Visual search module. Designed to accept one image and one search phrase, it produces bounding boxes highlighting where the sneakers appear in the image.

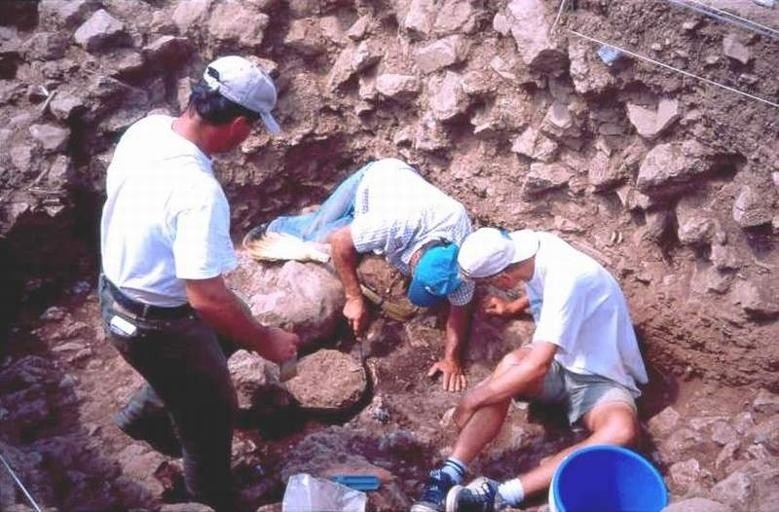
[241,222,278,255]
[118,396,184,458]
[445,474,504,512]
[408,467,459,512]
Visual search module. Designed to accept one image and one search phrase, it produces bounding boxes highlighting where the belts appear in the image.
[102,274,195,320]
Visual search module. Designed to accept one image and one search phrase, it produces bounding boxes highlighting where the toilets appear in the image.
[548,445,669,512]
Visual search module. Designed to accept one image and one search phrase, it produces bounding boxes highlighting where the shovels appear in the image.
[350,330,364,367]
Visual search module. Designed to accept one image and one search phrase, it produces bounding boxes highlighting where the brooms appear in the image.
[250,230,332,266]
[359,283,420,324]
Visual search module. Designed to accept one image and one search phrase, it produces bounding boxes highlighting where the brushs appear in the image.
[279,322,298,383]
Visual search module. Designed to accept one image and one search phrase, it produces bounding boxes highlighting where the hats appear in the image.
[457,226,539,279]
[407,242,463,307]
[202,55,282,134]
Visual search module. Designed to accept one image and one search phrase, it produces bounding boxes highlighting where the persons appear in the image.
[100,53,298,509]
[412,228,649,512]
[263,156,473,391]
